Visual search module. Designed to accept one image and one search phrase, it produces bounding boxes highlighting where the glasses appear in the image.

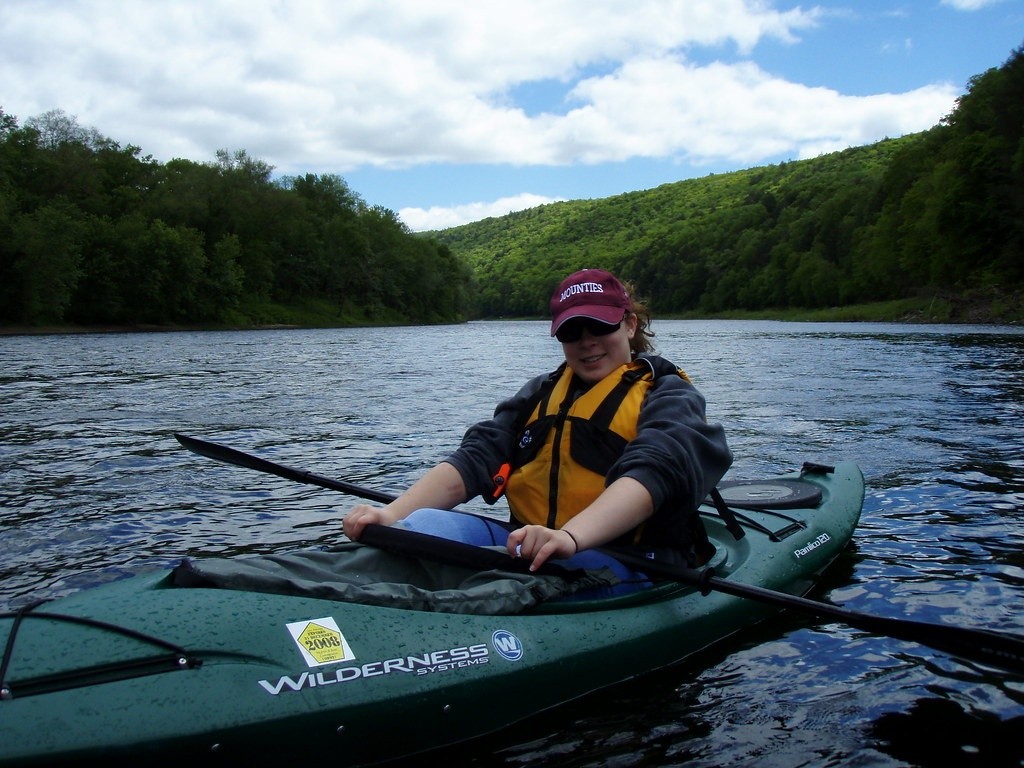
[553,318,626,344]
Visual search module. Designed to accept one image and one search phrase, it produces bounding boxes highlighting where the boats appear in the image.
[1,454,870,768]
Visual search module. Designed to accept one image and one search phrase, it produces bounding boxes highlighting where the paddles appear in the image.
[172,431,1024,670]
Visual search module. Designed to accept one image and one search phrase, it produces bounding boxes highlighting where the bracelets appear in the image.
[561,530,578,556]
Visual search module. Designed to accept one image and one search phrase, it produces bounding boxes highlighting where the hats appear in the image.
[551,269,633,337]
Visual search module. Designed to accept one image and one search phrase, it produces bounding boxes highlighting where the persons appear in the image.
[342,268,734,597]
